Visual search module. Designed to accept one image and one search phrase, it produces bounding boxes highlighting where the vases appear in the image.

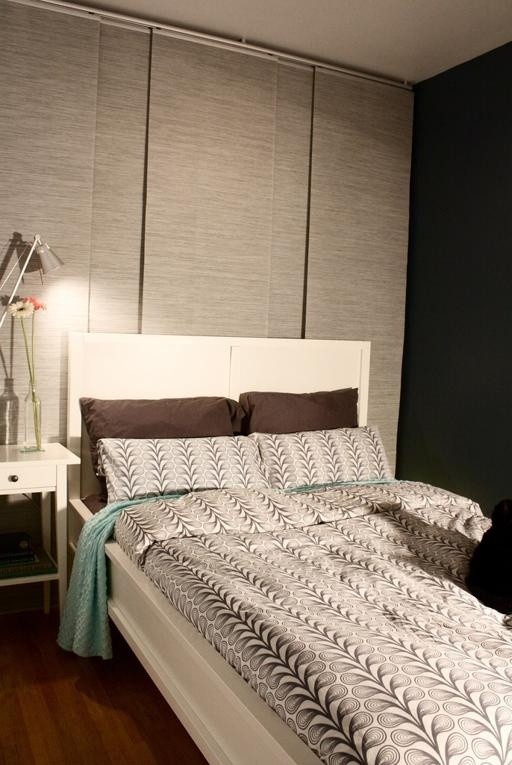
[18,381,41,452]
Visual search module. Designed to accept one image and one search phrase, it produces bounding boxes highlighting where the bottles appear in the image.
[20,380,42,452]
[0,378,18,445]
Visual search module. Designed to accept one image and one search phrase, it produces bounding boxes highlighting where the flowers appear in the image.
[9,297,47,450]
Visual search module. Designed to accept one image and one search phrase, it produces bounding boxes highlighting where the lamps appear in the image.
[0,234,64,329]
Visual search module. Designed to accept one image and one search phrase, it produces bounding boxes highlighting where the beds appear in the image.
[66,333,512,765]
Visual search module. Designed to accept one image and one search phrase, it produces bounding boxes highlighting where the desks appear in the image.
[0,443,82,624]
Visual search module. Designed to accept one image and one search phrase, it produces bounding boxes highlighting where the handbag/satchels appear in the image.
[466,498,512,614]
